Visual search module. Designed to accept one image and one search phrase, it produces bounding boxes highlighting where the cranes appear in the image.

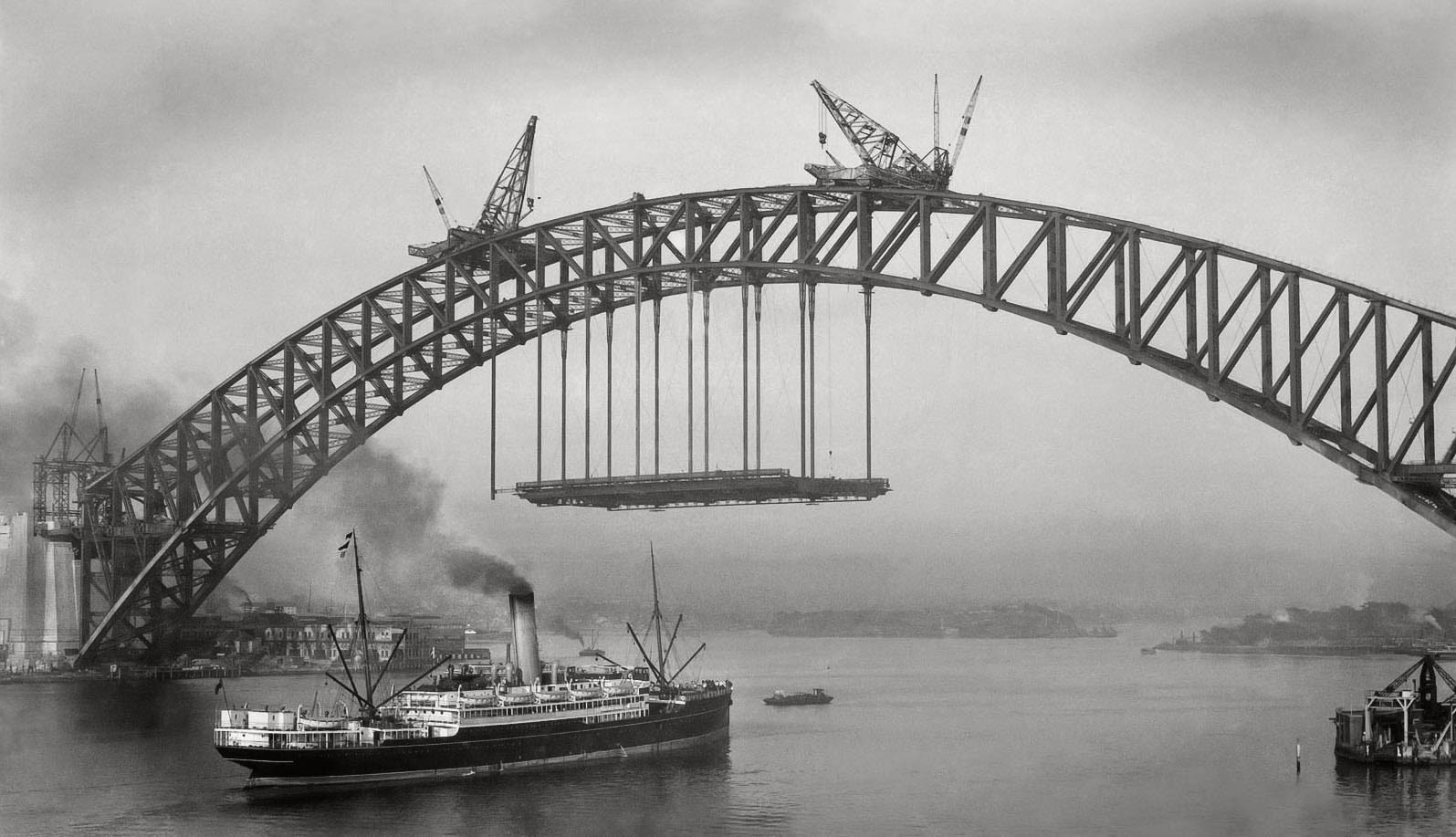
[33,368,117,527]
[407,116,562,277]
[804,72,983,212]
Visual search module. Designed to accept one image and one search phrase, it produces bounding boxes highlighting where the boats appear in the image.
[764,690,834,706]
[212,526,732,789]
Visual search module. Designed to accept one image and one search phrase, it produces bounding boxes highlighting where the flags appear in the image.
[215,677,222,695]
[338,540,349,550]
[345,531,352,538]
